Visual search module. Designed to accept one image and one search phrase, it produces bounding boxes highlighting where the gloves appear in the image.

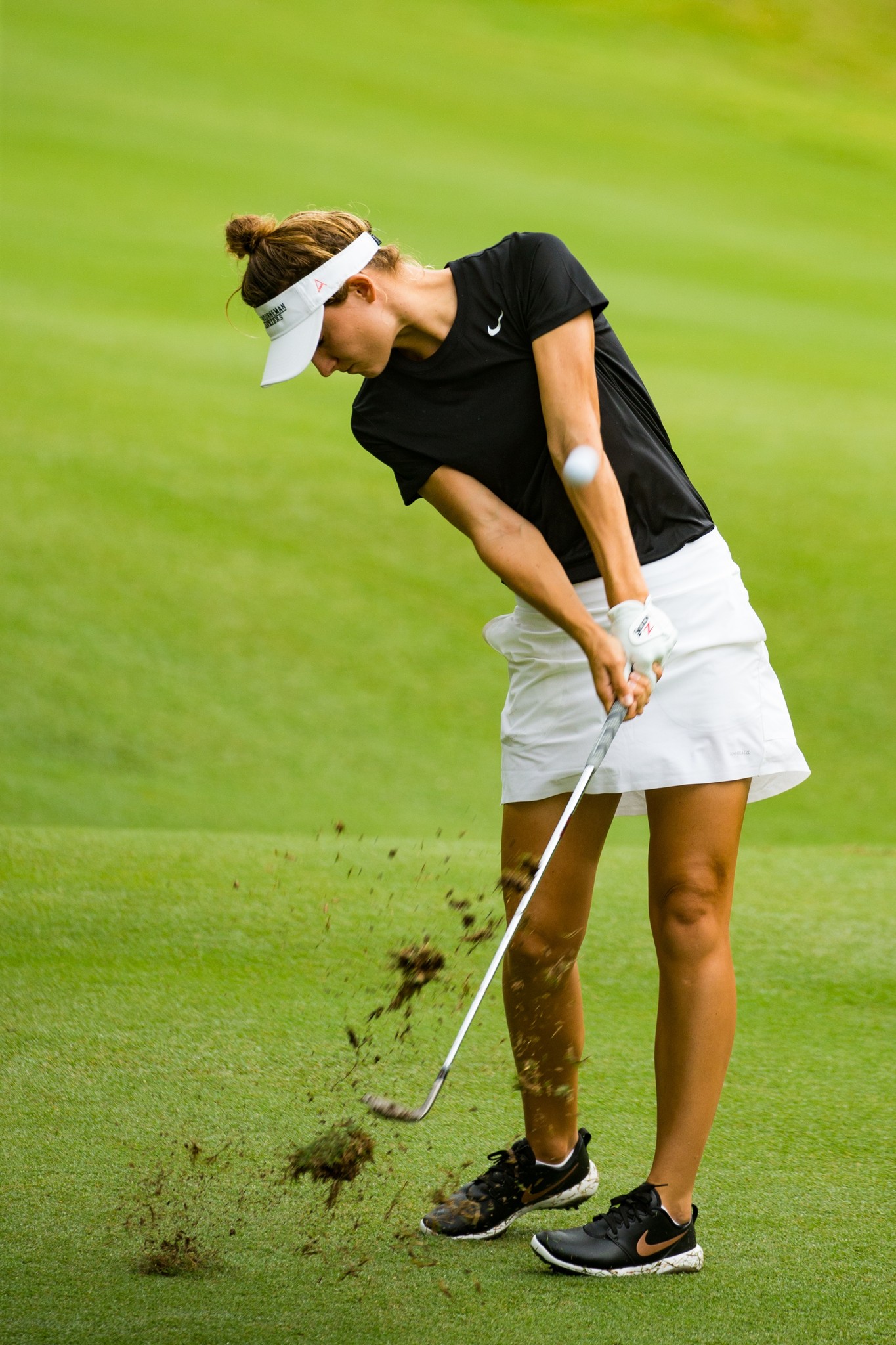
[607,600,664,692]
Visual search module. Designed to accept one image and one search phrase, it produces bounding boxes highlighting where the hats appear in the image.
[254,231,382,388]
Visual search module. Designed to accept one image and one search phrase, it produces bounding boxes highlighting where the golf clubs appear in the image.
[365,658,637,1123]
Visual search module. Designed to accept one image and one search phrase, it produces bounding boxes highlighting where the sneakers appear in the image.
[530,1182,705,1278]
[420,1126,599,1241]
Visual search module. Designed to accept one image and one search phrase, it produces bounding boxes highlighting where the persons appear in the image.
[224,203,808,1279]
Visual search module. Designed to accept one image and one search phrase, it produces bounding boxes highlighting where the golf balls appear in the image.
[564,444,602,485]
